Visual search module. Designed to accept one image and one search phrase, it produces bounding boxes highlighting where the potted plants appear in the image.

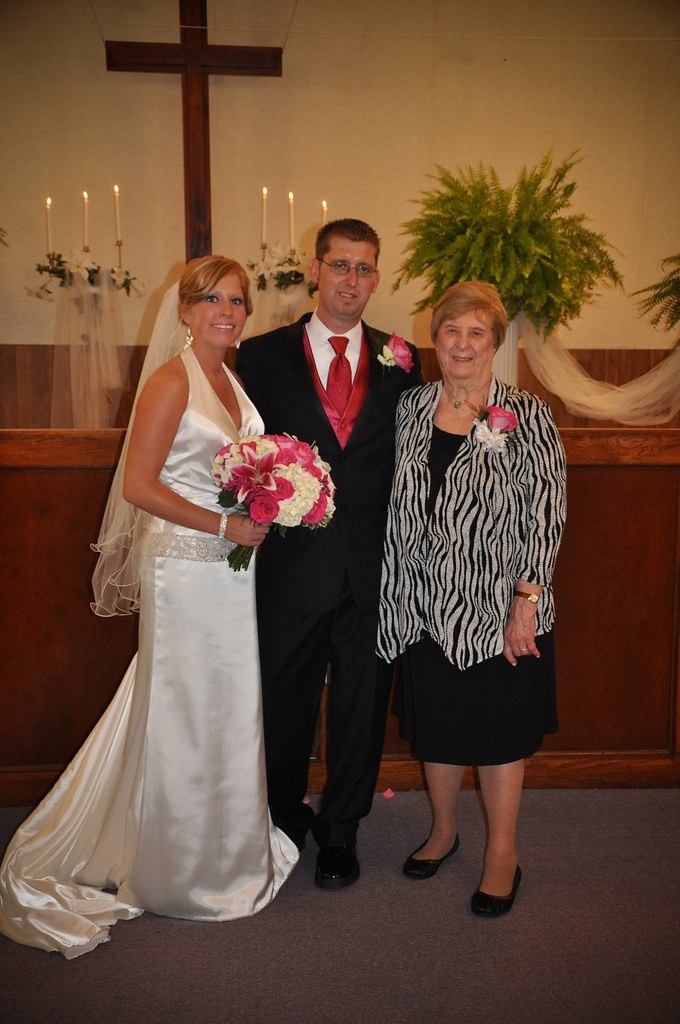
[388,146,628,385]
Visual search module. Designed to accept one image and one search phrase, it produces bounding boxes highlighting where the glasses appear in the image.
[318,258,377,277]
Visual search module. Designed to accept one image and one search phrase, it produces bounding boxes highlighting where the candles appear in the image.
[115,193,121,242]
[262,195,267,243]
[289,200,295,244]
[83,198,89,248]
[45,205,52,253]
[322,207,327,225]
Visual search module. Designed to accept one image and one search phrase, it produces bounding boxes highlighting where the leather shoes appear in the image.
[470,858,522,917]
[278,820,305,853]
[313,842,359,889]
[403,829,461,879]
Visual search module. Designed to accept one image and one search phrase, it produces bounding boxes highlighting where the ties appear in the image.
[325,336,351,418]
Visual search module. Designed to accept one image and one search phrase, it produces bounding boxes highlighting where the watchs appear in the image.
[514,591,540,603]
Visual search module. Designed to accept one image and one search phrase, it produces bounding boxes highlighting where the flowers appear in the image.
[210,433,337,572]
[463,398,523,457]
[376,332,415,376]
[248,243,319,299]
[23,252,147,303]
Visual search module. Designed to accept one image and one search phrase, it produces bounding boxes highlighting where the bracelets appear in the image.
[218,513,227,539]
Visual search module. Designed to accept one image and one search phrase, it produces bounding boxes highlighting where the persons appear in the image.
[0,255,301,959]
[376,280,565,915]
[237,218,421,887]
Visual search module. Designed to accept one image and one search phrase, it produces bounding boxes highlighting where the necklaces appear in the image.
[444,379,491,407]
